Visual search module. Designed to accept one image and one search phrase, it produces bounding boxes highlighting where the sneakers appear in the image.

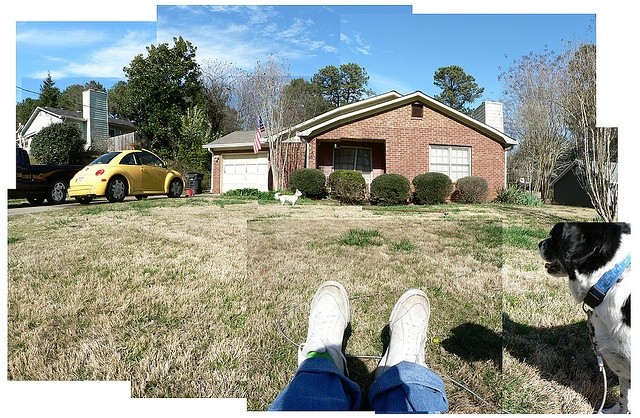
[373,289,431,378]
[297,281,350,376]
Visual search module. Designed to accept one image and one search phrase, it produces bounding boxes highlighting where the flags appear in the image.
[252,115,265,154]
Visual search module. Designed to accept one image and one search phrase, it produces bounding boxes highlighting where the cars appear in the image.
[67,148,184,203]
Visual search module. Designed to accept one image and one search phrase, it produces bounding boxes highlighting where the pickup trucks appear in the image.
[8,148,84,204]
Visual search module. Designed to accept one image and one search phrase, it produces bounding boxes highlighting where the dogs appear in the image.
[538,221,631,414]
[274,188,303,206]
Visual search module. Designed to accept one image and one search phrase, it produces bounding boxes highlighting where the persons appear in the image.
[267,280,450,414]
